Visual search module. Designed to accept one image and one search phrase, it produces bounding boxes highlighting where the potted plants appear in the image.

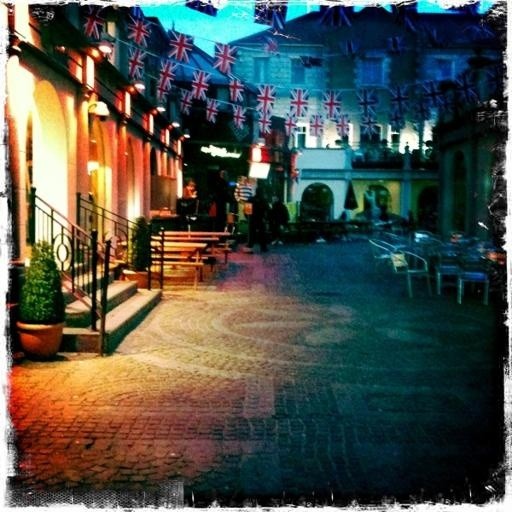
[122,216,152,289]
[16,240,65,363]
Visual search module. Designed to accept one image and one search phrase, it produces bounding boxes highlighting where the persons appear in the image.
[203,176,239,243]
[235,175,255,221]
[244,189,268,253]
[183,177,197,200]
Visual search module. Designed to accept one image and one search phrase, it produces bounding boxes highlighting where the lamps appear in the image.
[87,38,196,143]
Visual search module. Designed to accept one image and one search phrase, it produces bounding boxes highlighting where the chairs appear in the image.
[365,220,500,308]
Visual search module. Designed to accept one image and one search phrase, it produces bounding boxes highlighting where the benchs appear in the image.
[121,231,233,287]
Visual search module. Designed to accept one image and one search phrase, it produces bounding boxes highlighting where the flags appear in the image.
[309,89,349,136]
[356,72,478,140]
[185,1,496,55]
[483,66,500,94]
[129,48,309,136]
[85,5,238,75]
